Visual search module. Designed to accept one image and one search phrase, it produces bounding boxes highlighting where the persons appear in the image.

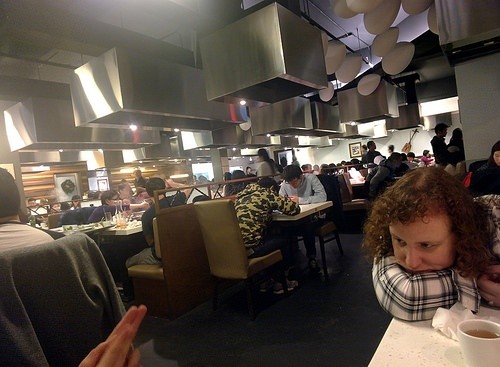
[117,177,186,291]
[235,177,300,294]
[446,128,465,162]
[0,166,68,259]
[26,136,434,215]
[84,189,150,224]
[359,165,500,321]
[79,304,148,367]
[275,164,327,275]
[430,123,450,166]
[463,140,500,197]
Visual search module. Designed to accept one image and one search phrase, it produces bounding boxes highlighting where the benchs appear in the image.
[127,195,241,319]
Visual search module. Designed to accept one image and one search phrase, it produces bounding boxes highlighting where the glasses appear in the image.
[109,196,119,201]
[285,175,300,184]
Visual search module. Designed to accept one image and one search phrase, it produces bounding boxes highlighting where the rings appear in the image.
[487,299,496,307]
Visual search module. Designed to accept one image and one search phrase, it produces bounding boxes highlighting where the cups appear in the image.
[104,212,112,222]
[116,214,127,229]
[457,319,500,367]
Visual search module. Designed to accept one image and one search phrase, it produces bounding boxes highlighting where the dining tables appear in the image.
[368,302,500,367]
[27,218,142,267]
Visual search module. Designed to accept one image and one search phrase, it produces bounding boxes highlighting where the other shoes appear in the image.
[306,252,317,263]
[260,280,298,294]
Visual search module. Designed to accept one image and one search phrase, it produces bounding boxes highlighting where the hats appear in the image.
[374,156,385,165]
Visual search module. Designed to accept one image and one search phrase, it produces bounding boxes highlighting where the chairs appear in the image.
[194,199,288,321]
[0,232,135,367]
[470,160,489,174]
[338,173,370,234]
[286,174,343,277]
[33,206,98,229]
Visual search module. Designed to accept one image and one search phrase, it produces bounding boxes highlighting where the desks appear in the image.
[349,179,365,200]
[272,201,333,282]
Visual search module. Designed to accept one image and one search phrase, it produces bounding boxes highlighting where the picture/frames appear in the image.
[349,142,362,158]
